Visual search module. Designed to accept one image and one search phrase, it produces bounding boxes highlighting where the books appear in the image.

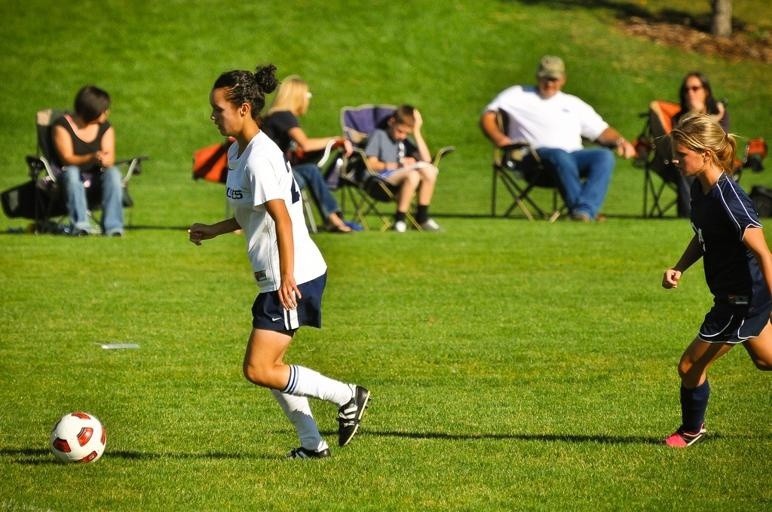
[380,162,439,179]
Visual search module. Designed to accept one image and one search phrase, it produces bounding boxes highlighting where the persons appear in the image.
[480,55,636,224]
[50,87,125,237]
[672,71,729,217]
[188,63,371,460]
[259,75,355,234]
[663,113,772,448]
[365,106,441,233]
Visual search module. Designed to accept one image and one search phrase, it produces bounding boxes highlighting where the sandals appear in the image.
[328,224,352,235]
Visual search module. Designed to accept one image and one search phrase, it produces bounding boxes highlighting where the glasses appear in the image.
[680,84,702,93]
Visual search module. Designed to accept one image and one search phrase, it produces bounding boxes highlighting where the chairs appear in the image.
[632,100,728,219]
[338,103,456,233]
[490,107,587,224]
[26,107,150,238]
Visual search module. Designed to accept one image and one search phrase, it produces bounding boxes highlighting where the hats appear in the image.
[535,54,564,79]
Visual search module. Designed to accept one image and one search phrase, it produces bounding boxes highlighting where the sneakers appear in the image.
[338,384,370,447]
[285,447,331,460]
[571,211,606,224]
[395,218,441,233]
[665,422,708,448]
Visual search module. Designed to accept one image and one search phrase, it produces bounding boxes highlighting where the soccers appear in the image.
[49,412,106,466]
